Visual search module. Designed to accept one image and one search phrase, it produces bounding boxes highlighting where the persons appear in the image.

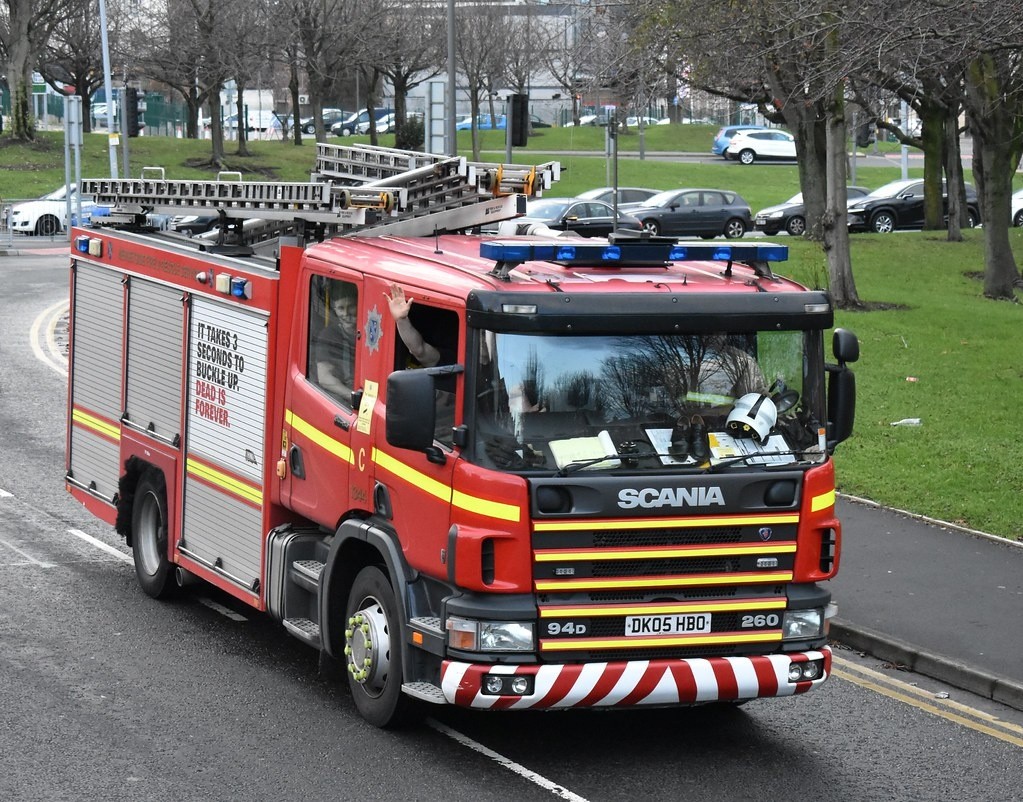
[383,282,548,412]
[660,330,774,415]
[316,288,357,402]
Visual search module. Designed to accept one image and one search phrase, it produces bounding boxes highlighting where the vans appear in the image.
[329,108,397,138]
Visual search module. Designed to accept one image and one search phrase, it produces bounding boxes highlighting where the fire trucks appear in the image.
[63,144,860,731]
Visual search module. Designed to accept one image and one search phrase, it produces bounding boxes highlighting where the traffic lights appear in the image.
[855,112,876,148]
[120,83,146,137]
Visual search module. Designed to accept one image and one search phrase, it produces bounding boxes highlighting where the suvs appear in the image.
[726,129,798,165]
[712,125,786,161]
[619,188,753,240]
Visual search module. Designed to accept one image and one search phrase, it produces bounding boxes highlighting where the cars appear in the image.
[656,117,712,126]
[7,183,96,236]
[754,185,871,237]
[300,111,355,134]
[1011,188,1023,227]
[530,114,553,129]
[202,110,296,131]
[456,114,506,132]
[357,111,425,136]
[68,204,112,228]
[576,187,662,216]
[563,115,614,127]
[465,198,645,237]
[847,178,983,235]
[618,117,659,128]
